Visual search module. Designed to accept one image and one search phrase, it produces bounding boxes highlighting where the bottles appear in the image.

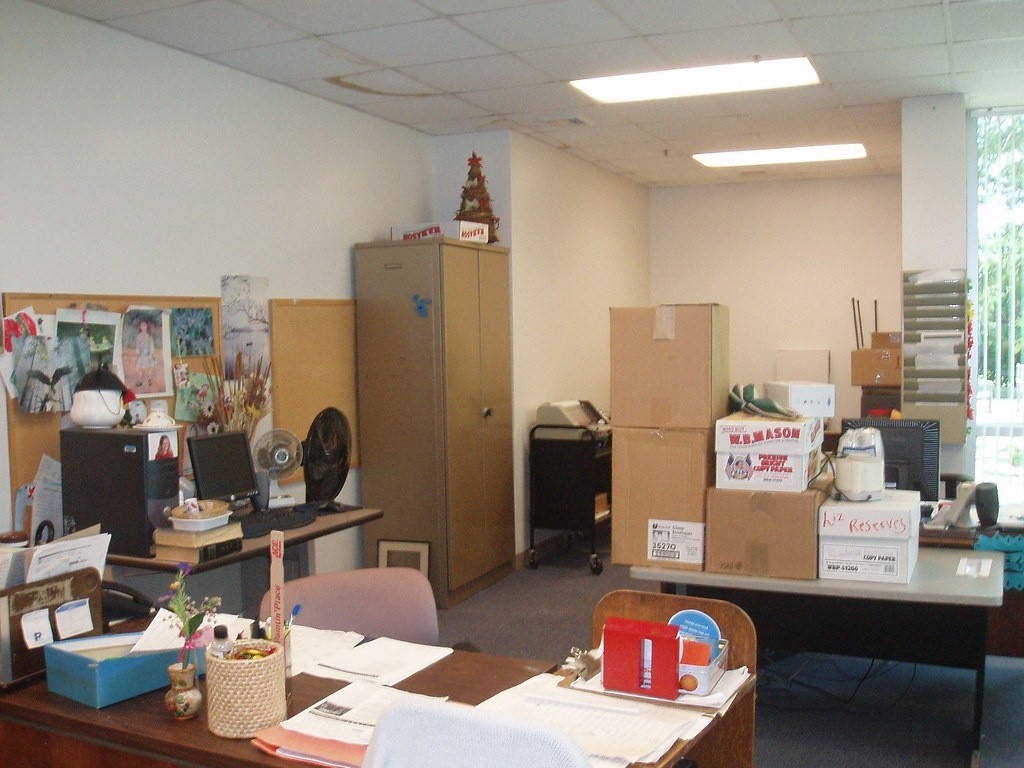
[68,390,127,429]
[206,625,234,654]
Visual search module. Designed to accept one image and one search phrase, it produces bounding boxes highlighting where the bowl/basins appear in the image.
[141,408,175,426]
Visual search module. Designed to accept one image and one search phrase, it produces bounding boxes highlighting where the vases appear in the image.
[165,663,203,721]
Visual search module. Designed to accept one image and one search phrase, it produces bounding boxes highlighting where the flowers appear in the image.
[149,563,224,667]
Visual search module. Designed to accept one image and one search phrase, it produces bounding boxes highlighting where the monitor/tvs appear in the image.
[187,429,260,502]
[841,419,939,502]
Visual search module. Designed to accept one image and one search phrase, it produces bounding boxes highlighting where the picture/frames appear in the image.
[377,538,431,582]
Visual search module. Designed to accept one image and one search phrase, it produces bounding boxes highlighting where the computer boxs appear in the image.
[59,427,182,558]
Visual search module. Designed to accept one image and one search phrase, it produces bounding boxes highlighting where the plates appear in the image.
[132,425,183,431]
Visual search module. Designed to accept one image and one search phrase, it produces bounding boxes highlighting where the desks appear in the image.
[630,546,1005,752]
[0,590,757,768]
[919,519,1024,662]
[104,506,381,619]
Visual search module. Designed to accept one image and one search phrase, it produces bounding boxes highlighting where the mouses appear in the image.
[316,502,345,513]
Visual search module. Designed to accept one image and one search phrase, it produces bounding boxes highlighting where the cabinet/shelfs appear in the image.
[528,423,611,573]
[357,237,516,607]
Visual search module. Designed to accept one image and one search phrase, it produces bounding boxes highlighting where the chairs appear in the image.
[258,566,439,645]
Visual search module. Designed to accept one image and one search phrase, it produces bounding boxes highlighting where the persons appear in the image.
[153,435,173,460]
[132,317,156,387]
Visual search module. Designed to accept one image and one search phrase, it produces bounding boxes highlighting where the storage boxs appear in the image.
[404,221,488,243]
[608,301,920,584]
[45,632,205,709]
[595,491,609,515]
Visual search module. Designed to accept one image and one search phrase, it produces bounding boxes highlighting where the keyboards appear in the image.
[233,505,315,538]
[921,505,934,517]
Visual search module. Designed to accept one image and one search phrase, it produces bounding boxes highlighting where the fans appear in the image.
[295,407,353,512]
[254,429,304,509]
